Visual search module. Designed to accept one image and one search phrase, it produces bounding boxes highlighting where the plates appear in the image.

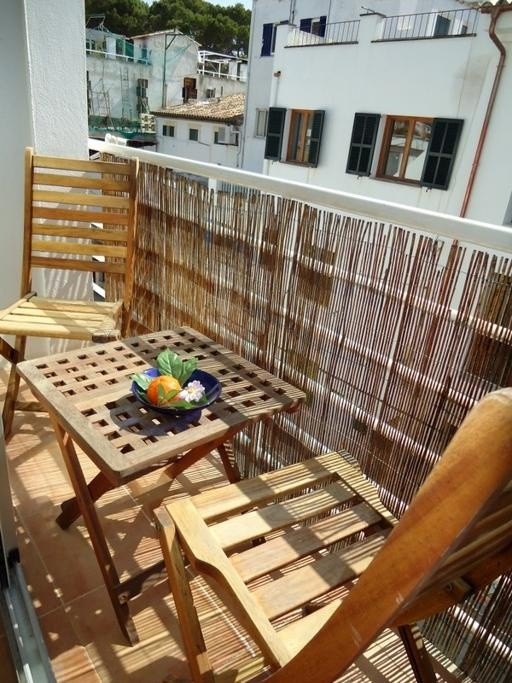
[132,369,222,417]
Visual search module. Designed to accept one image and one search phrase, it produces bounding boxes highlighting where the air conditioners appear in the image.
[218,125,236,144]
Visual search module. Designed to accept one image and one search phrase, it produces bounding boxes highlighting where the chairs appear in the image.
[152,387,511,682]
[1,146,139,441]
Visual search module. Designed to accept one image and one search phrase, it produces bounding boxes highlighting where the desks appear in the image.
[15,325,306,649]
